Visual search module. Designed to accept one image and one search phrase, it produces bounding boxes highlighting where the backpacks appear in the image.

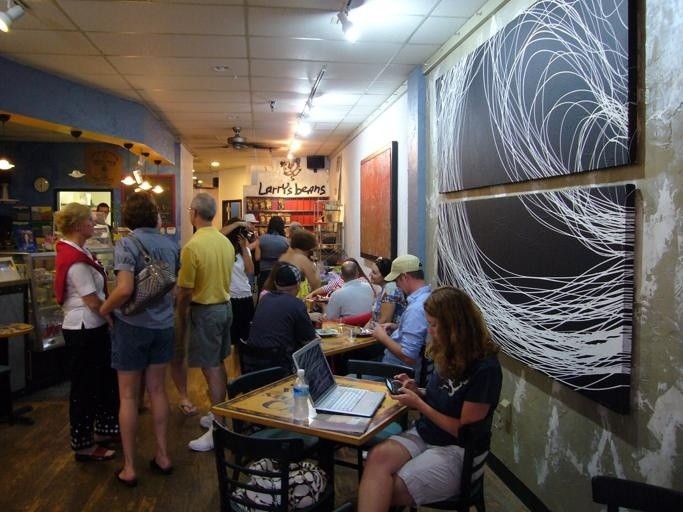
[233,457,330,510]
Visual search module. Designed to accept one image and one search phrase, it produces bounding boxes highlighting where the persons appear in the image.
[324,261,382,326]
[254,216,288,283]
[280,230,322,293]
[244,263,320,387]
[57,204,121,461]
[342,254,436,383]
[361,256,406,362]
[98,193,181,485]
[258,261,288,303]
[219,217,255,378]
[290,220,305,232]
[95,203,113,234]
[356,286,502,512]
[174,193,235,451]
[306,277,346,301]
[241,213,260,231]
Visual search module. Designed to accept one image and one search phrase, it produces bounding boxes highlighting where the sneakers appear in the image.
[200,413,222,428]
[189,426,215,453]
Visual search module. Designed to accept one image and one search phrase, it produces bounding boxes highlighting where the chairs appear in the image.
[227,366,288,487]
[590,474,683,512]
[423,432,490,512]
[210,417,336,511]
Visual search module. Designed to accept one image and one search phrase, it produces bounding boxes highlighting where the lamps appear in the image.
[337,12,359,39]
[153,160,164,194]
[139,153,152,191]
[121,144,136,187]
[1,2,22,34]
[68,131,86,178]
[0,113,15,170]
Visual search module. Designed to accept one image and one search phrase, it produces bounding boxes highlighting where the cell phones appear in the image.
[385,376,405,395]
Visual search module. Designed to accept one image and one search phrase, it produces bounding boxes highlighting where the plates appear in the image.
[314,328,340,336]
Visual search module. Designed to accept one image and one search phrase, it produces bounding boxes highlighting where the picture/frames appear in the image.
[359,141,398,261]
[121,174,176,231]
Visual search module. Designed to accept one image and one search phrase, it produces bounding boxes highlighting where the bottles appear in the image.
[294,369,309,418]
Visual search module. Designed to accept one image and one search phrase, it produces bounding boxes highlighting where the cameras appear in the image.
[242,227,254,240]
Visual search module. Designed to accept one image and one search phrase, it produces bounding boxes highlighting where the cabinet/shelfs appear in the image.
[245,195,330,235]
[1,247,116,352]
[314,200,346,269]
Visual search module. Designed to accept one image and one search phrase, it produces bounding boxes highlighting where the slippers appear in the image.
[74,446,116,461]
[149,457,173,475]
[116,467,136,488]
[178,399,198,417]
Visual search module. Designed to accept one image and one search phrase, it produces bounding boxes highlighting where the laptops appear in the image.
[292,337,386,419]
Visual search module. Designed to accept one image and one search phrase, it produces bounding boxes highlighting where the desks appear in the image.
[1,323,35,426]
[210,374,410,485]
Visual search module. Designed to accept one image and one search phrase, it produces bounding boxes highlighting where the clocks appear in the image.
[34,178,50,192]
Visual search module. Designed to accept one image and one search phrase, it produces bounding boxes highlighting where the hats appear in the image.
[384,255,422,282]
[376,256,392,277]
[242,214,259,223]
[275,265,301,286]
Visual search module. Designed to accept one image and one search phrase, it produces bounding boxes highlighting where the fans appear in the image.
[214,127,284,149]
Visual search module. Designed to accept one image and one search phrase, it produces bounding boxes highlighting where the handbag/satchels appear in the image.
[120,233,175,317]
